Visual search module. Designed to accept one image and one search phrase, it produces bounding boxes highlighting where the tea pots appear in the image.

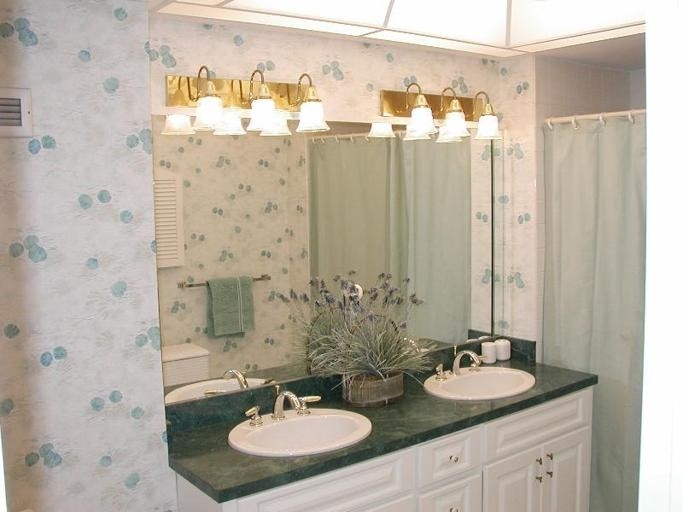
[150,114,495,406]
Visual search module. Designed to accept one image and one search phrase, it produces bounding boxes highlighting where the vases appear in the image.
[307,270,436,387]
[276,271,379,376]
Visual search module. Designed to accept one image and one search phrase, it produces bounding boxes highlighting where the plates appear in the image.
[417,422,483,512]
[484,382,594,512]
[221,445,418,512]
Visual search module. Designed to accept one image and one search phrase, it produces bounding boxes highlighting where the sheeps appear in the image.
[452,350,481,375]
[272,390,301,419]
[223,370,250,390]
[397,336,421,356]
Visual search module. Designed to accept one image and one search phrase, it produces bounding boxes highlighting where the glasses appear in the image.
[474,91,503,140]
[435,127,463,143]
[402,125,432,141]
[295,73,331,133]
[161,115,195,135]
[246,70,278,131]
[193,66,224,132]
[367,122,396,137]
[440,87,471,138]
[213,118,247,136]
[259,119,292,136]
[406,82,438,136]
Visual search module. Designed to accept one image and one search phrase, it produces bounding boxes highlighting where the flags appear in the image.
[342,379,404,407]
